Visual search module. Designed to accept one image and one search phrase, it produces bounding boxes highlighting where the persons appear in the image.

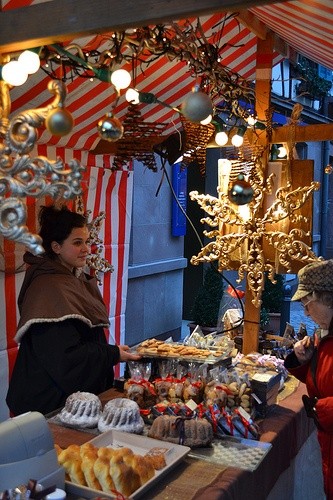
[5,205,144,416]
[285,260,333,499]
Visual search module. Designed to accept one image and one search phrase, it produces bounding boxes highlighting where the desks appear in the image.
[43,381,319,500]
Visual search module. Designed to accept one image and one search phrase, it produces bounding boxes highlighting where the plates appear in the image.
[64,429,191,500]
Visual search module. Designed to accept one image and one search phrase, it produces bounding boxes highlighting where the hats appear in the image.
[291,259,333,302]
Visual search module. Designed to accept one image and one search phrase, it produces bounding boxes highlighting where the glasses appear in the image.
[301,299,313,311]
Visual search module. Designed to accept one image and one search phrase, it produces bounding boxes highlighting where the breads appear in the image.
[54,443,166,497]
[58,338,276,447]
